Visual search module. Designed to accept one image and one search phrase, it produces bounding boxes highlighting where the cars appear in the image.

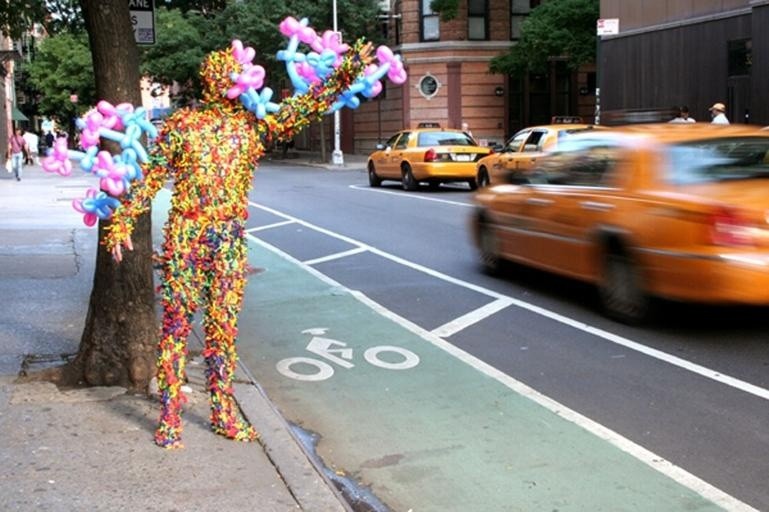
[468,122,769,327]
[475,117,609,191]
[367,122,494,191]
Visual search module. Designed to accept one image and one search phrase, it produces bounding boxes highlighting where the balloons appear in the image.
[226,16,409,122]
[42,97,160,227]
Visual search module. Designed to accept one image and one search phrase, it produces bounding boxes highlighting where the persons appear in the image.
[4,126,79,182]
[679,105,698,123]
[96,34,379,453]
[665,105,688,123]
[460,123,472,137]
[708,103,731,124]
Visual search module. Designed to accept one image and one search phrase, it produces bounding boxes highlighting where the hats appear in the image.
[707,102,726,112]
